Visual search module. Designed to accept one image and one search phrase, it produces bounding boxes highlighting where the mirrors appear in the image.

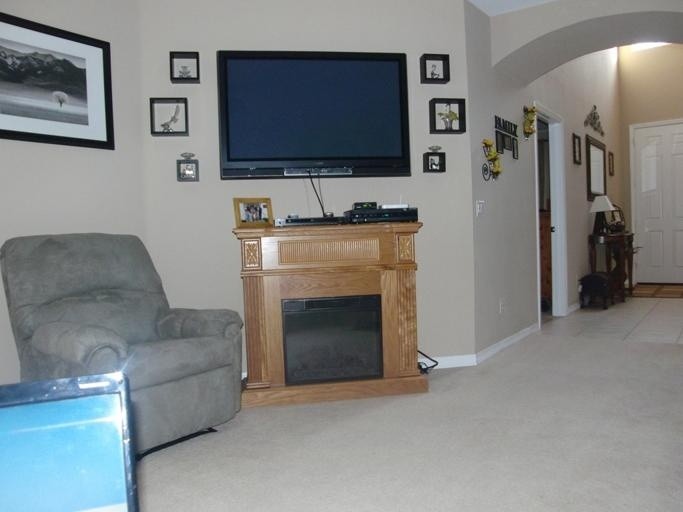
[585,134,608,202]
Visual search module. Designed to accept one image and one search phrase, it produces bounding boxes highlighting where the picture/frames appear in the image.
[495,130,519,159]
[429,97,466,134]
[573,133,581,165]
[609,151,614,176]
[0,12,115,150]
[169,51,200,80]
[150,97,188,134]
[177,160,199,182]
[233,197,274,228]
[423,151,446,173]
[419,53,450,84]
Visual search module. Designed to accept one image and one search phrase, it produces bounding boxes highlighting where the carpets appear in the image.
[628,283,682,299]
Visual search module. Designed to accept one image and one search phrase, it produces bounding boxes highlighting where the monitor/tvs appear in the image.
[216,49,411,181]
[0,371,139,512]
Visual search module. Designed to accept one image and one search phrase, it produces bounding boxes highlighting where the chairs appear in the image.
[0,233,244,461]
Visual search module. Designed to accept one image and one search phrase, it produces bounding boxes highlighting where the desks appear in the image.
[233,222,429,408]
[588,232,634,302]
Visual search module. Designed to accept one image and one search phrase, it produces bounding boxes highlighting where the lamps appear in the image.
[589,195,616,234]
